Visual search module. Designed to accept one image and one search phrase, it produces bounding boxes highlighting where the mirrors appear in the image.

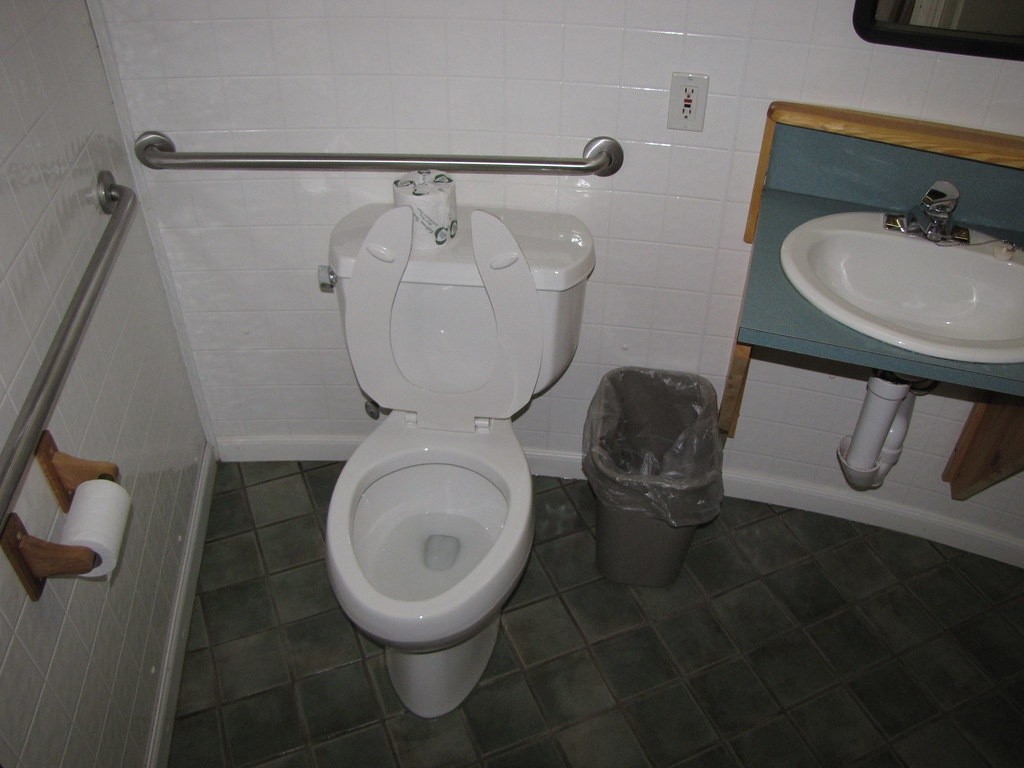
[852,0,1024,62]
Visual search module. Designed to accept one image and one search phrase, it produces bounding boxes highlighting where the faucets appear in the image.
[880,179,972,248]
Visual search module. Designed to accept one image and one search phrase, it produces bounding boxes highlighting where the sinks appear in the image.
[778,210,1024,363]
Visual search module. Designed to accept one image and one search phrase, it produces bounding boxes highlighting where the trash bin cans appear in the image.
[595,365,717,586]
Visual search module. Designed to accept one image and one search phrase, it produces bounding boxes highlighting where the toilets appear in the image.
[318,200,597,721]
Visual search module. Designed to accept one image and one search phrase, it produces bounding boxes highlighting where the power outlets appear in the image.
[667,72,710,132]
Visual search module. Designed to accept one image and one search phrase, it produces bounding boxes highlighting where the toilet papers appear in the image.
[391,169,459,254]
[61,477,131,579]
